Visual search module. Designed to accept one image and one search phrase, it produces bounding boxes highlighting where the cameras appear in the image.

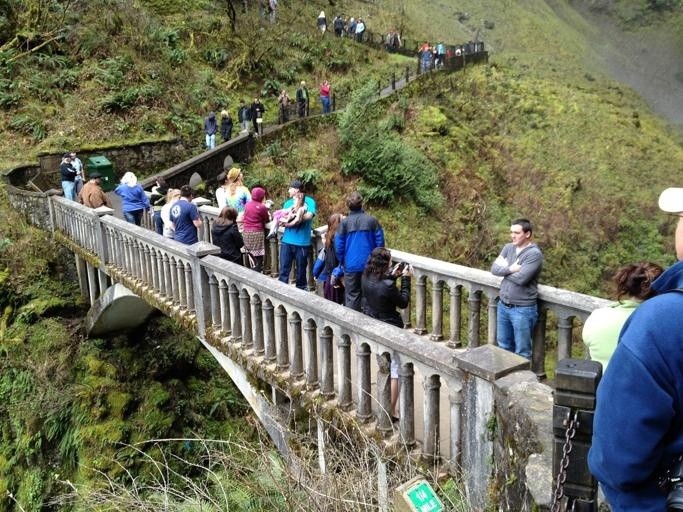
[398,261,408,270]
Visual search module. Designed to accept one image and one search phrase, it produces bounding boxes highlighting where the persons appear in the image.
[237,99,252,133]
[295,80,310,117]
[113,171,203,245]
[385,31,394,52]
[491,218,542,371]
[582,261,663,376]
[59,149,115,216]
[250,99,265,133]
[587,185,682,511]
[360,247,412,423]
[389,33,403,54]
[219,110,233,143]
[319,79,331,115]
[312,10,365,44]
[277,88,292,125]
[417,40,454,73]
[205,168,386,308]
[203,110,218,150]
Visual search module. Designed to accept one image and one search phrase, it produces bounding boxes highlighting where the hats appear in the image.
[227,167,241,182]
[291,181,307,193]
[658,187,683,213]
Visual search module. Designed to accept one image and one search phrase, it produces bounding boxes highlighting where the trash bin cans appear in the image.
[86,155,113,192]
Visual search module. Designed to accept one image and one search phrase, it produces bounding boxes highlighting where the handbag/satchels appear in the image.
[242,253,259,269]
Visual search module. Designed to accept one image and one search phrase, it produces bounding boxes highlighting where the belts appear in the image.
[499,299,519,308]
